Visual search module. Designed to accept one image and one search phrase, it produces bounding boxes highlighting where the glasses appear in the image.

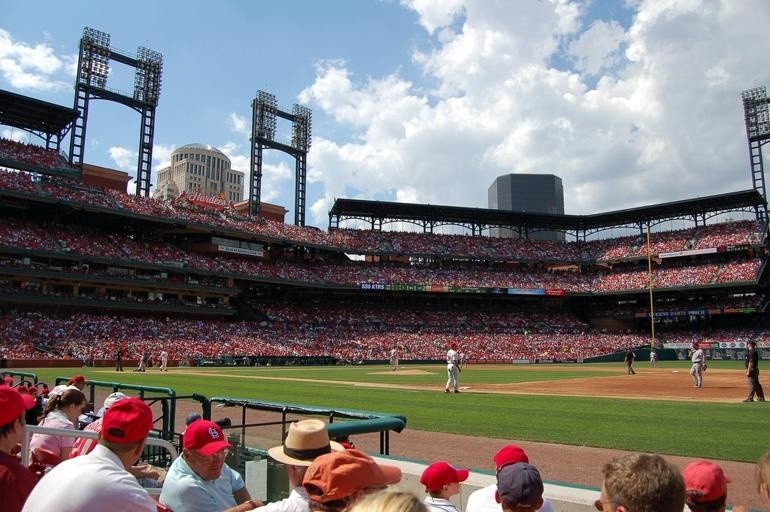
[595,500,629,512]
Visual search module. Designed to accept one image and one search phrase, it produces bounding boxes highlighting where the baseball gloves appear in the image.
[701,364,707,371]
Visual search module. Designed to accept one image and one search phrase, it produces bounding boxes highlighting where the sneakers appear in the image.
[743,398,753,402]
[454,390,459,393]
[445,389,450,392]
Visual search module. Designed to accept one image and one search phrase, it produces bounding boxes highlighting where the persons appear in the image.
[390,345,399,371]
[649,350,656,368]
[115,348,123,372]
[160,348,169,372]
[0,135,770,361]
[624,348,635,375]
[0,375,552,512]
[742,341,765,402]
[594,449,770,512]
[445,343,461,393]
[138,348,147,373]
[689,342,707,387]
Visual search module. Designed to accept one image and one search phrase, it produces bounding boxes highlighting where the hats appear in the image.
[0,384,36,428]
[103,392,130,408]
[183,418,232,457]
[682,461,731,503]
[102,398,154,443]
[492,445,528,469]
[2,375,85,396]
[497,460,544,510]
[451,343,458,348]
[420,462,468,494]
[302,448,402,503]
[267,419,345,466]
